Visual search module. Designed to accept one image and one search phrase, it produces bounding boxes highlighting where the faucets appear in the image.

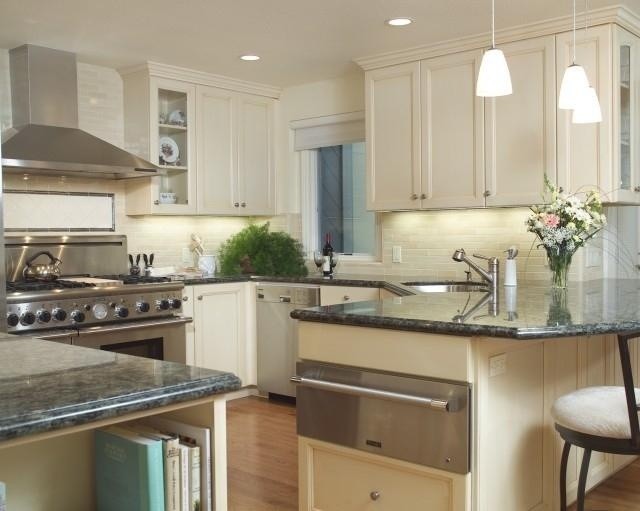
[453,289,498,321]
[452,248,500,288]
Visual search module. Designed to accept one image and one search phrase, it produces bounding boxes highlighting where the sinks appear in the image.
[401,280,490,293]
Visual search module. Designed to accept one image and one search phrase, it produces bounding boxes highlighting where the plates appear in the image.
[169,111,184,124]
[159,135,179,163]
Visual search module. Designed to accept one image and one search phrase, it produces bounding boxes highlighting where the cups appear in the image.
[314,251,322,275]
[332,253,338,276]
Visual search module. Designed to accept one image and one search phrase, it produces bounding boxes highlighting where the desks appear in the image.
[293,276,640,511]
[1,329,242,510]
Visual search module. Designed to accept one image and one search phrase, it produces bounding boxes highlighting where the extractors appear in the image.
[1,45,167,179]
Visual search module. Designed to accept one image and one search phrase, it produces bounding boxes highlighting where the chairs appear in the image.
[551,328,640,509]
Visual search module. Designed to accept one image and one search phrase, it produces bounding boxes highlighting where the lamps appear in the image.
[474,0,514,97]
[574,0,604,125]
[558,2,590,111]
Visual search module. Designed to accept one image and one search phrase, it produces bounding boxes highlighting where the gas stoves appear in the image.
[3,234,193,365]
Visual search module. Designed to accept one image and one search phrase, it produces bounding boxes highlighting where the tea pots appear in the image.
[22,250,64,281]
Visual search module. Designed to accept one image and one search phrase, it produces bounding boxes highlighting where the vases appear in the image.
[547,245,573,329]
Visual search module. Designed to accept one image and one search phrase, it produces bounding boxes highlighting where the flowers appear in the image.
[527,174,608,271]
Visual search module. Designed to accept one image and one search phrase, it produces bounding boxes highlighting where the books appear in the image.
[96,418,214,511]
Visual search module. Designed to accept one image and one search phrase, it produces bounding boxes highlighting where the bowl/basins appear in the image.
[159,193,179,202]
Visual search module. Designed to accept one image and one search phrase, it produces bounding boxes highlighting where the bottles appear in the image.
[323,232,333,274]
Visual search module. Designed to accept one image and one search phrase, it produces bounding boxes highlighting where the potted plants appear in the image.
[218,222,309,278]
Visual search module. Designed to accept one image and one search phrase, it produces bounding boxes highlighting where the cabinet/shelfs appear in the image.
[485,21,613,208]
[366,48,485,211]
[255,280,318,399]
[610,22,639,204]
[199,84,282,218]
[184,285,255,391]
[120,73,196,216]
